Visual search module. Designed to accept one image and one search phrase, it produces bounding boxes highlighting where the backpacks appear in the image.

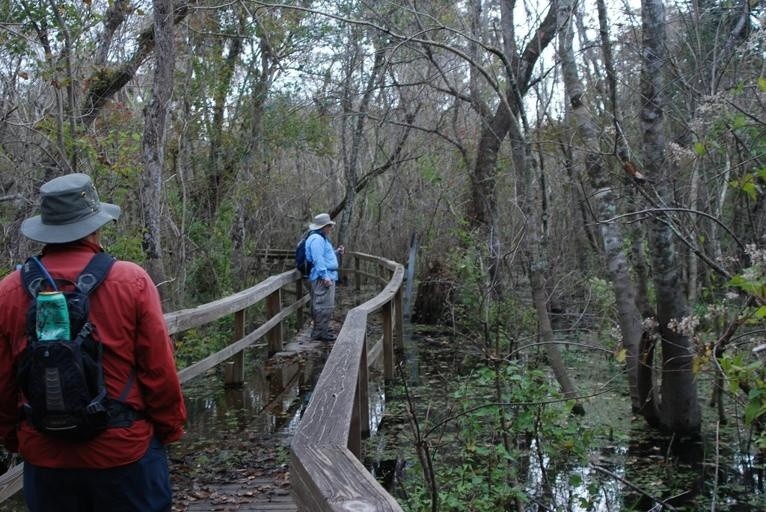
[294,231,326,279]
[10,252,151,448]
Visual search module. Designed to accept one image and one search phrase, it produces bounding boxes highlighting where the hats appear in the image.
[309,214,336,230]
[20,172,121,244]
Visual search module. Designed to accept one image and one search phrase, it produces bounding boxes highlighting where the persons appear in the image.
[0,173,187,512]
[305,213,344,340]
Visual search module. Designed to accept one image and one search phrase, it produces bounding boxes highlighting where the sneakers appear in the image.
[316,332,337,341]
